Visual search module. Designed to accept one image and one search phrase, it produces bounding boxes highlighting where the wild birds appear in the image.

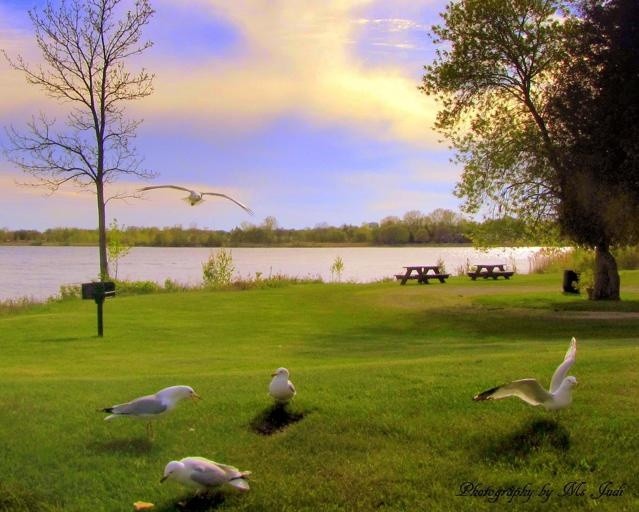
[268,366,297,407]
[94,384,202,440]
[473,336,579,421]
[160,456,253,499]
[136,184,253,213]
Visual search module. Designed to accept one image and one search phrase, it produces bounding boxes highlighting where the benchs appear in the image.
[394,272,451,285]
[466,270,516,280]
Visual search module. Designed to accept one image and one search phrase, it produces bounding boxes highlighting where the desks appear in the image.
[472,264,508,281]
[401,265,447,284]
